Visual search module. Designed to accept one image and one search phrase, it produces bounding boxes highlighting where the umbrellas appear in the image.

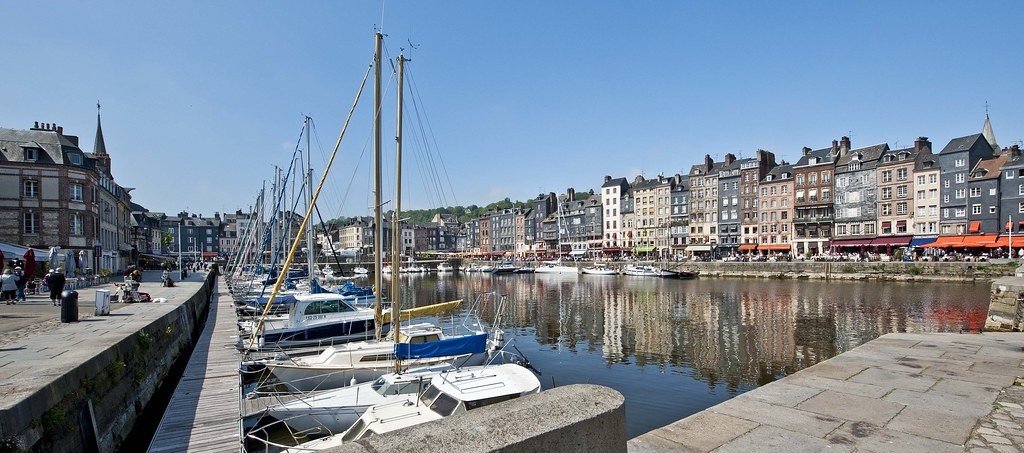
[78,250,87,275]
[49,246,61,268]
[23,249,35,280]
[65,249,76,278]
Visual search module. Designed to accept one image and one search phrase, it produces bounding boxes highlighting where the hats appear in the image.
[133,270,141,275]
[3,269,13,274]
[55,267,64,274]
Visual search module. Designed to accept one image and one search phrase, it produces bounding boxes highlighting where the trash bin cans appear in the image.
[61,290,78,322]
[182,269,187,278]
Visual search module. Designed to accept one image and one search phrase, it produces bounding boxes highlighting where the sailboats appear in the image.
[460,190,657,275]
[660,215,678,277]
[223,0,511,395]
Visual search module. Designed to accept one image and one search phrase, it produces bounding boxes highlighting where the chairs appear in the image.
[31,274,108,293]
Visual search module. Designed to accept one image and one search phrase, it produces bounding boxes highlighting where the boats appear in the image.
[679,267,700,278]
[245,337,543,453]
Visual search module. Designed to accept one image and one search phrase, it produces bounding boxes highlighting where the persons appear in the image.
[0,265,66,307]
[123,265,141,287]
[160,256,209,288]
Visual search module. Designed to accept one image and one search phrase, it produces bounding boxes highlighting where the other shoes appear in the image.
[10,301,16,305]
[18,298,27,303]
[57,304,62,306]
[52,304,57,306]
[6,301,11,305]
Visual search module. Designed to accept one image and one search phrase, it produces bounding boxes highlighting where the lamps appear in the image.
[95,201,112,214]
